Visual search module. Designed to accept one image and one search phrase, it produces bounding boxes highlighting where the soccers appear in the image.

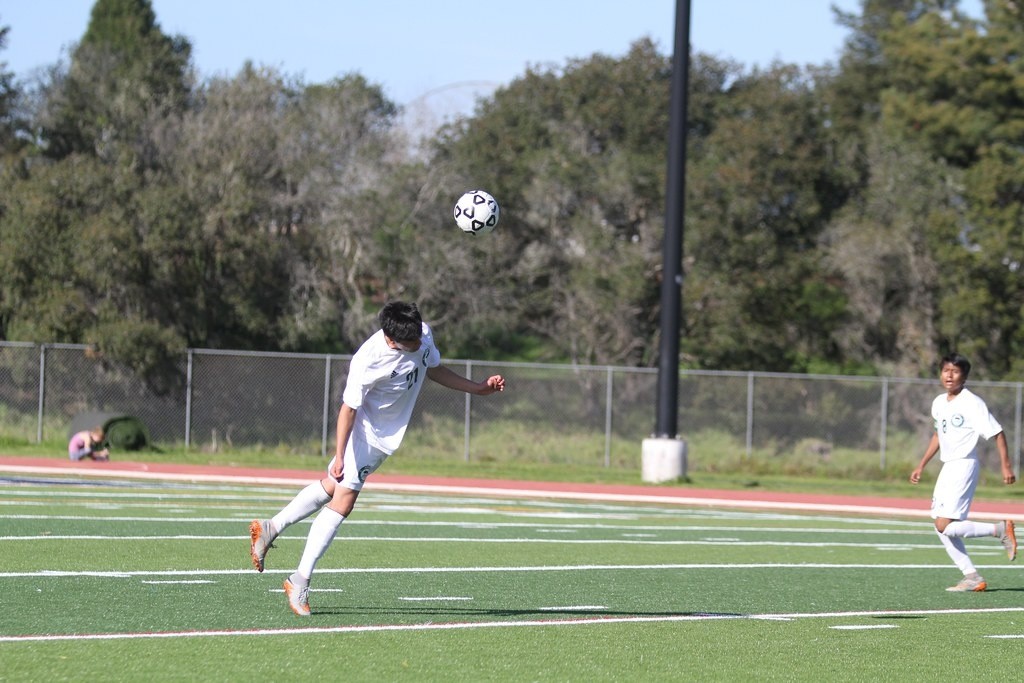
[453,190,500,238]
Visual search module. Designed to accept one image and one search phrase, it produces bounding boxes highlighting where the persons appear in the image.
[909,354,1017,592]
[69,427,109,462]
[250,301,507,616]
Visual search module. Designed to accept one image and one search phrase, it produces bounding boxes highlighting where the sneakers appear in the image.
[284,573,311,616]
[250,519,276,572]
[999,520,1017,561]
[945,571,986,591]
